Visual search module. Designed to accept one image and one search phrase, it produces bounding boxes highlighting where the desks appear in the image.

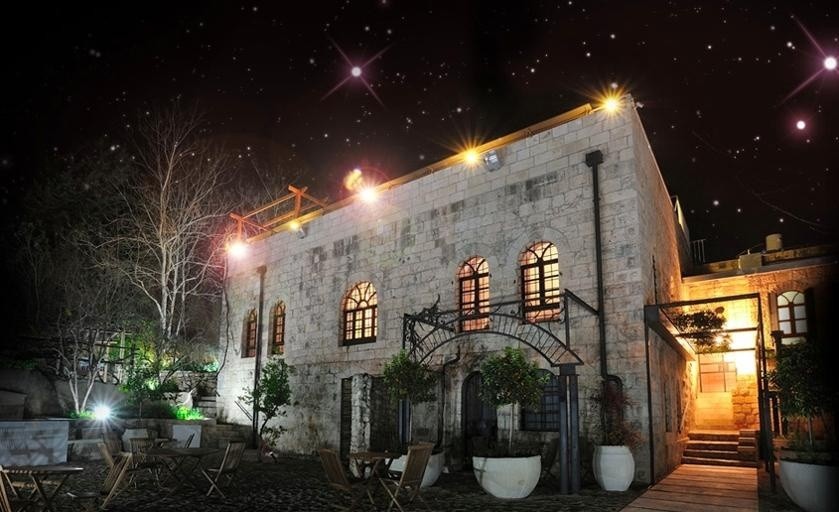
[4,468,83,511]
[347,452,399,511]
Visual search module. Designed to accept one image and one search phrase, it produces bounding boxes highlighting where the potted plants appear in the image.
[764,342,839,511]
[581,375,645,491]
[473,346,551,499]
[237,345,297,487]
[162,358,207,411]
[384,348,448,488]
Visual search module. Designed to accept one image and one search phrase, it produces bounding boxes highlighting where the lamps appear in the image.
[484,150,502,171]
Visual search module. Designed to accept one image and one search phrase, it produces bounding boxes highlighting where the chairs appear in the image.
[377,446,432,512]
[317,450,375,511]
[385,440,435,503]
[0,466,33,511]
[83,433,247,512]
